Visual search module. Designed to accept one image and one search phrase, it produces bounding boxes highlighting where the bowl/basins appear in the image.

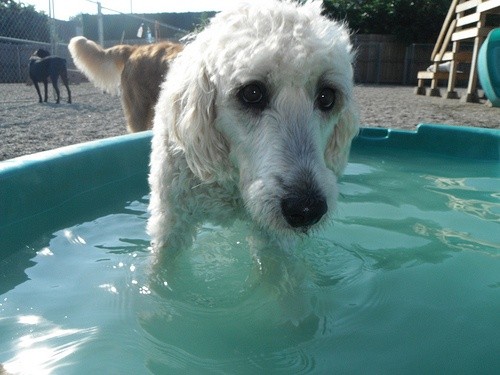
[478,27,500,107]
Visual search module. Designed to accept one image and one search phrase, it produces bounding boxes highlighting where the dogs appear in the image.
[147,0,360,283]
[68,35,185,134]
[28,48,71,105]
[427,60,465,74]
